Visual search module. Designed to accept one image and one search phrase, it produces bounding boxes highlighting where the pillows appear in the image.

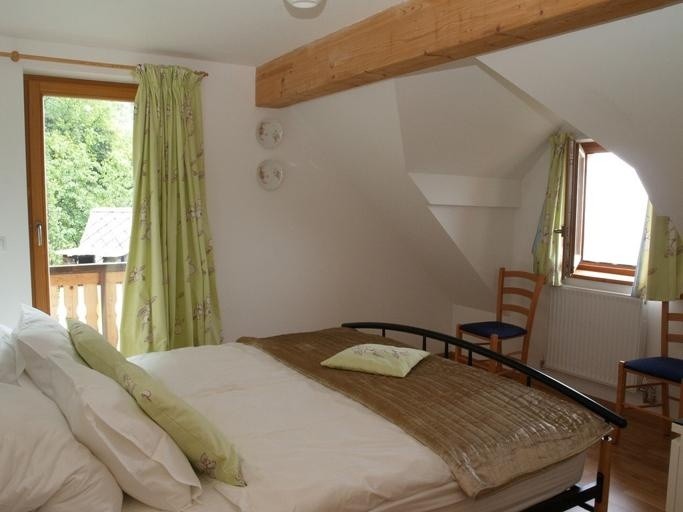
[65,314,247,490]
[16,303,202,512]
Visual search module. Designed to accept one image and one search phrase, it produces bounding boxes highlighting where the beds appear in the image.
[113,321,629,512]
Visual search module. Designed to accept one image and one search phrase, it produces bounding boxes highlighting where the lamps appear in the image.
[285,0,325,18]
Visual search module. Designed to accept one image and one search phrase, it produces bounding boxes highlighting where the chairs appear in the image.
[456,268,544,384]
[614,301,683,444]
